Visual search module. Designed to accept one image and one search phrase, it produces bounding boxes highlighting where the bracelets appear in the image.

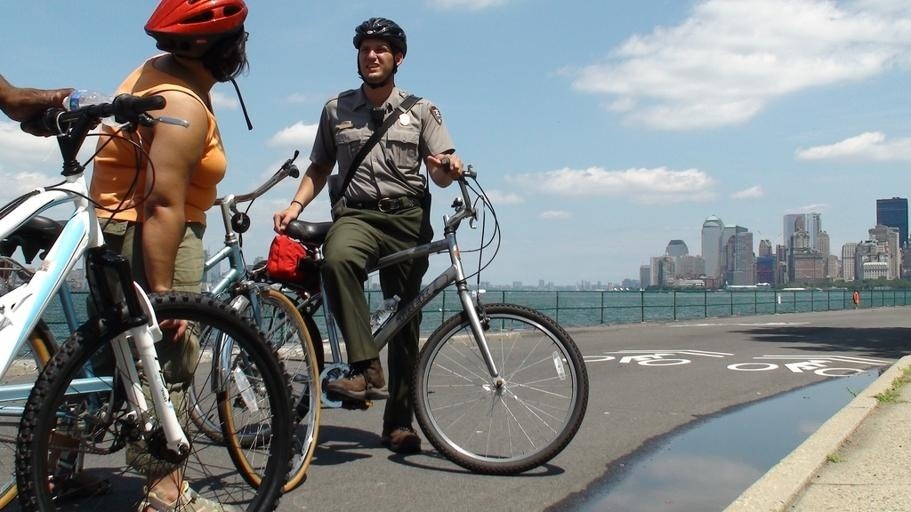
[291,201,304,212]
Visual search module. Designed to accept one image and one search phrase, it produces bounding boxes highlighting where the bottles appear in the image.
[369,295,402,335]
[65,88,107,114]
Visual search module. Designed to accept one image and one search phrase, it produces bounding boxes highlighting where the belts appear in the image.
[346,193,423,215]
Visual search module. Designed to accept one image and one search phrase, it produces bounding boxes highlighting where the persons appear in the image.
[274,17,463,452]
[0,73,84,123]
[86,0,250,512]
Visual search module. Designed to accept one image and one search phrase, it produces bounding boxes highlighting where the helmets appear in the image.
[144,0,249,55]
[351,17,408,57]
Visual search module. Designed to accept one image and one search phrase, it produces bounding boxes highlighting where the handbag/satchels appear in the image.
[265,230,318,290]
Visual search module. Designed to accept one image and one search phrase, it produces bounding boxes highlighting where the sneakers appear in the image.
[324,355,392,403]
[130,479,239,512]
[380,421,423,457]
